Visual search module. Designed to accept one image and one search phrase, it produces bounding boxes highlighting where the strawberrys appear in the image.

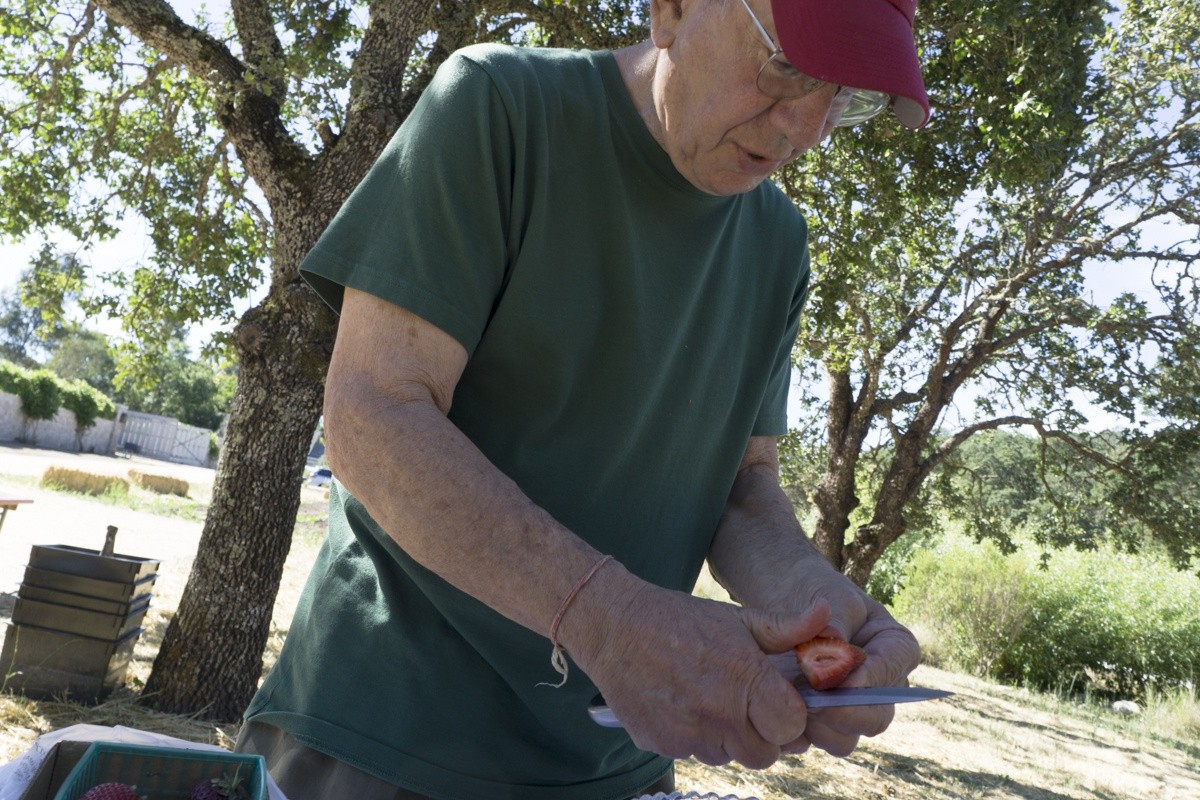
[80,772,250,800]
[794,637,866,690]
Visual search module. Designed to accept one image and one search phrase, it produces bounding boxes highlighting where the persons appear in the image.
[228,0,918,800]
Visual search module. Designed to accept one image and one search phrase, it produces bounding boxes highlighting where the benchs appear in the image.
[0,496,34,530]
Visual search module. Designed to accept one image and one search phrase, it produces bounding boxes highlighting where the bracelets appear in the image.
[535,554,614,690]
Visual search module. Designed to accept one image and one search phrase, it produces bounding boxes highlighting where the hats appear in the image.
[773,0,931,129]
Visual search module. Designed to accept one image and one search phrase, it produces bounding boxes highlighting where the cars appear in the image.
[309,467,333,488]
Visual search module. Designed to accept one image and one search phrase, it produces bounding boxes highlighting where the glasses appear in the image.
[740,0,890,129]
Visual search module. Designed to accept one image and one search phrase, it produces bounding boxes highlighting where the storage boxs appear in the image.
[0,544,160,707]
[18,740,270,800]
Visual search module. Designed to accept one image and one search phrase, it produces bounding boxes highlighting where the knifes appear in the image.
[586,684,954,729]
